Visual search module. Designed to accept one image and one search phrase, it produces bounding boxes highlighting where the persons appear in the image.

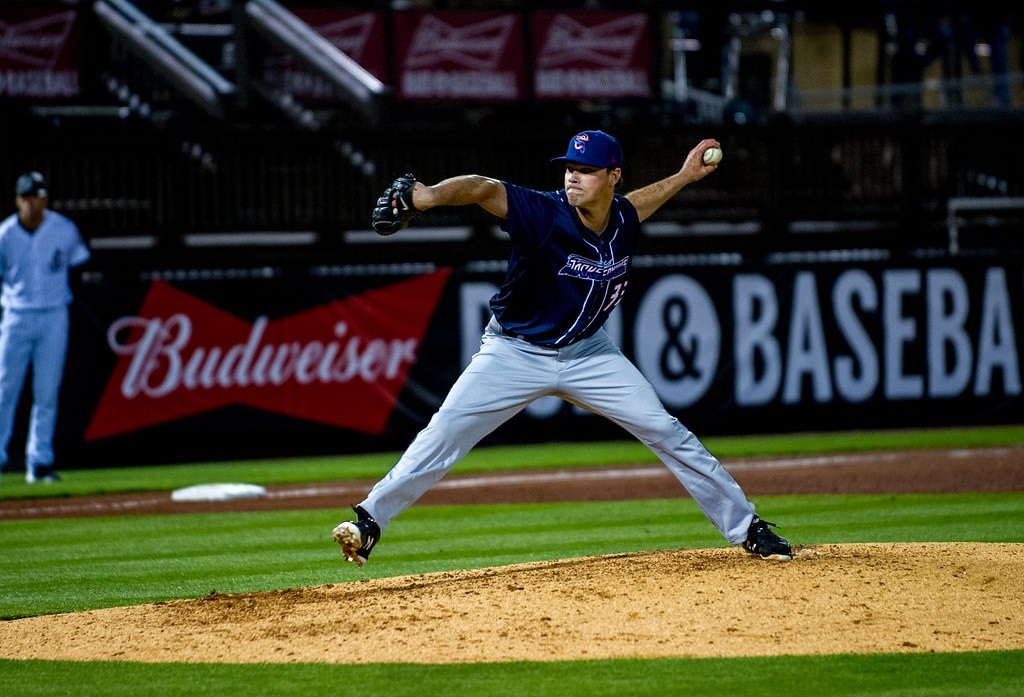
[333,131,793,566]
[0,172,90,482]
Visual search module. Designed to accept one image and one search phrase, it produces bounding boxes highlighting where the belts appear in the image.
[502,328,518,339]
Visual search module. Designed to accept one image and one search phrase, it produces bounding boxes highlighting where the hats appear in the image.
[549,129,623,169]
[13,172,48,197]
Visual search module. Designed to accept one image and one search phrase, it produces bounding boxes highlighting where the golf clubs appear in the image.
[373,173,417,237]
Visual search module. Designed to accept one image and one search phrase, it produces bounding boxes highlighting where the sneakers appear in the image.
[739,513,793,561]
[328,503,382,565]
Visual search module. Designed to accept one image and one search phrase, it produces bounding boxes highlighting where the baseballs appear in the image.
[703,147,723,166]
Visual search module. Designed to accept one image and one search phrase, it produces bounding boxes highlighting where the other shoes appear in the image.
[24,470,61,483]
